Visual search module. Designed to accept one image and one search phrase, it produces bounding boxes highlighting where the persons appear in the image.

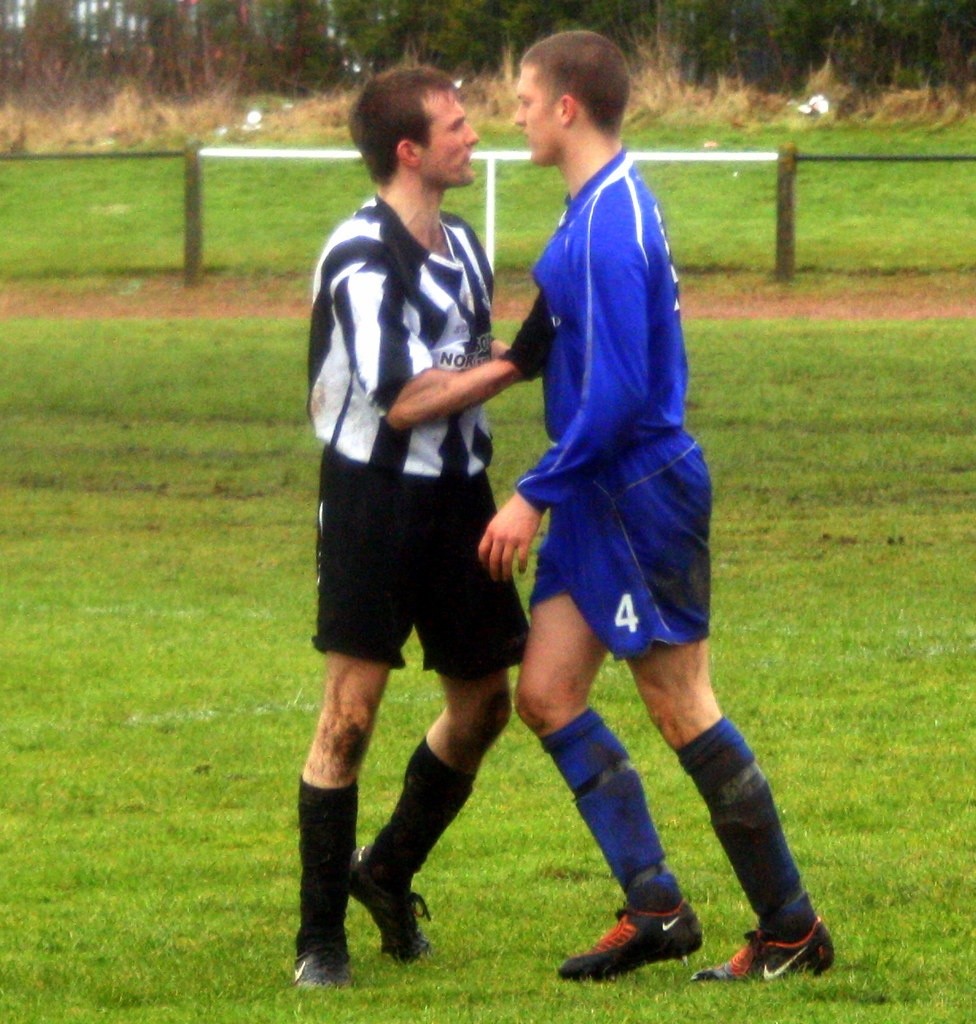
[477,32,834,984]
[282,64,554,990]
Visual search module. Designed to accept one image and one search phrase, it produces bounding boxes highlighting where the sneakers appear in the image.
[291,925,356,991]
[556,895,703,982]
[347,845,435,965]
[691,917,835,985]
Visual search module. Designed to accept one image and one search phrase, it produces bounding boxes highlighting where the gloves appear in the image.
[500,293,553,379]
